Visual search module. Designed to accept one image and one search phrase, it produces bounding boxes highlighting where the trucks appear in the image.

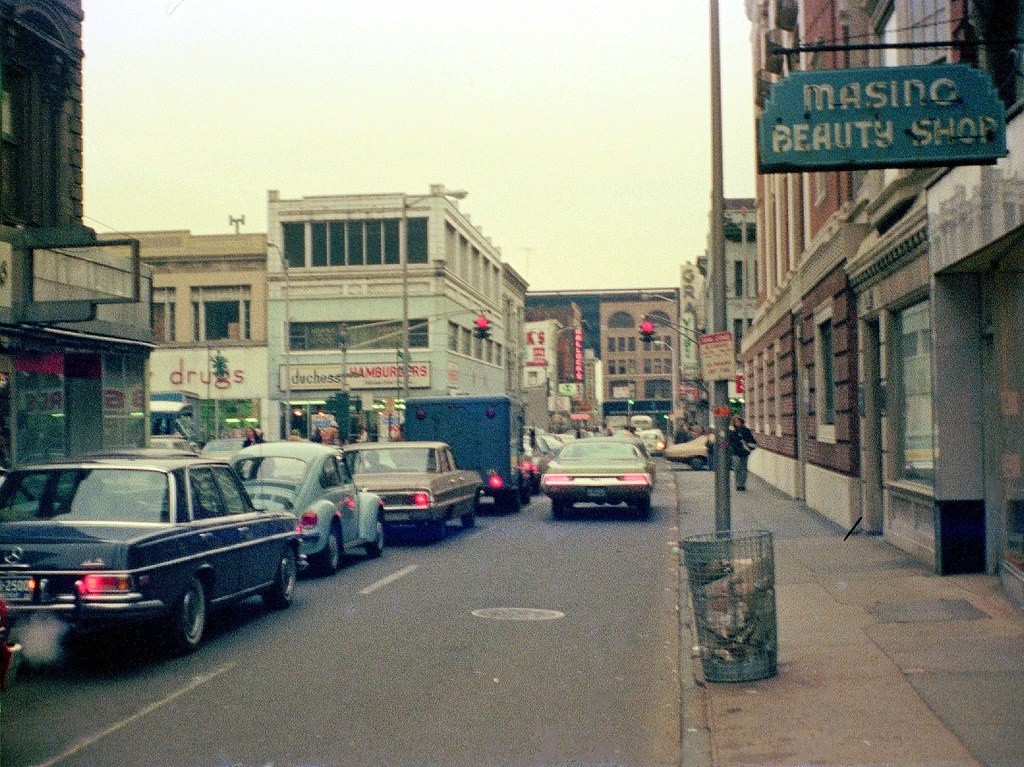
[405,391,525,514]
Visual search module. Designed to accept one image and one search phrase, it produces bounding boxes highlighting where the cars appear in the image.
[85,433,253,489]
[541,437,655,520]
[223,441,384,577]
[524,415,666,494]
[666,432,736,473]
[339,439,486,543]
[1,445,308,658]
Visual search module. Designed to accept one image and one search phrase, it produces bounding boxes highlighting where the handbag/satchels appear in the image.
[742,442,756,452]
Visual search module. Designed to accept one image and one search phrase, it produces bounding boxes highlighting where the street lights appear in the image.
[401,188,468,397]
[265,239,292,441]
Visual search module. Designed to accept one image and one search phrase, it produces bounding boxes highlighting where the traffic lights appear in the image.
[473,320,484,339]
[482,319,492,338]
[648,322,656,341]
[638,321,649,342]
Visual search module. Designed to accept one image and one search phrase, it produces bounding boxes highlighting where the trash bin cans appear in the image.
[682,531,782,684]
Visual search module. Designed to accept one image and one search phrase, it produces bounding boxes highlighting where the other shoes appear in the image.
[736,486,745,490]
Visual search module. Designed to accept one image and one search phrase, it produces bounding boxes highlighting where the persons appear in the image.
[354,428,368,442]
[309,427,323,444]
[729,415,756,491]
[546,422,705,445]
[242,427,267,448]
